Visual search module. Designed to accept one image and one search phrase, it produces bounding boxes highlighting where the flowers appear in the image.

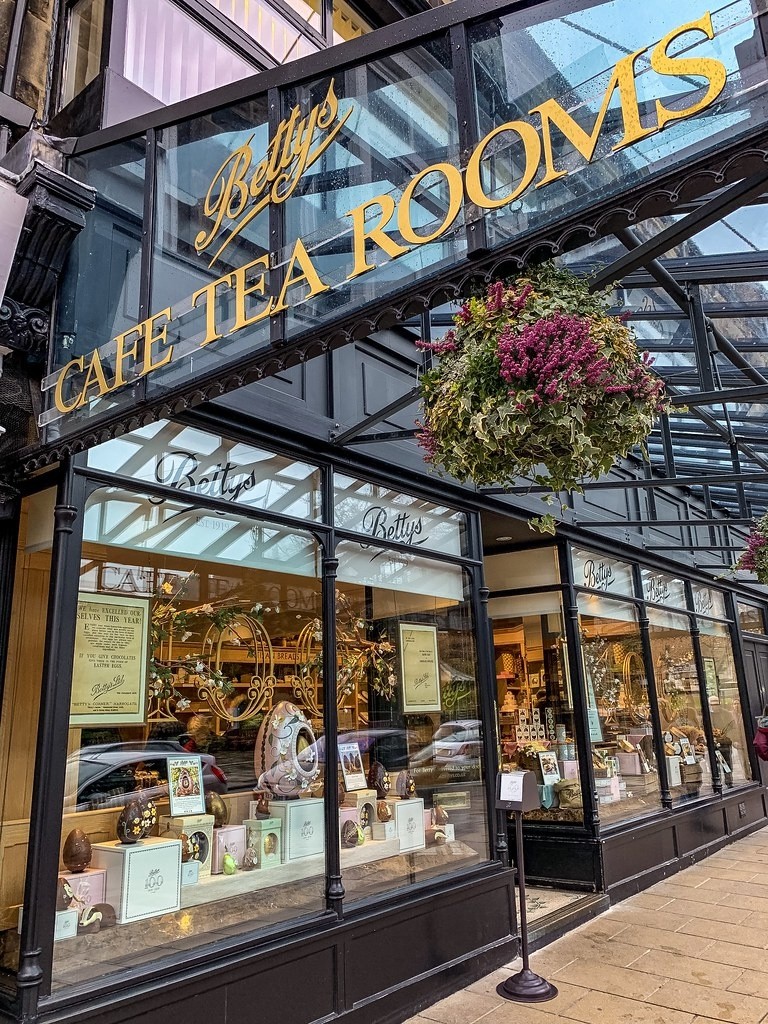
[414,263,671,532]
[715,513,768,583]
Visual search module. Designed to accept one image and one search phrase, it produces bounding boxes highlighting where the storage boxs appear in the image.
[508,666,568,744]
[541,728,704,805]
[17,789,455,943]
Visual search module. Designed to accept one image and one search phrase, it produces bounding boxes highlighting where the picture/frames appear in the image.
[394,620,443,715]
[68,587,151,729]
[702,656,721,706]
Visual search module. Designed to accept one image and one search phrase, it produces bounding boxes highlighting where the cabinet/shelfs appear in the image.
[119,640,359,750]
